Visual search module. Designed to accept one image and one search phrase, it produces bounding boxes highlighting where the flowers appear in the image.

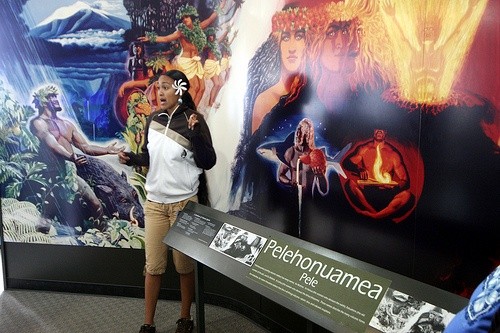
[172,79,187,96]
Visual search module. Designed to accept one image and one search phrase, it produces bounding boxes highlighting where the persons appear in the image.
[119,70,217,333]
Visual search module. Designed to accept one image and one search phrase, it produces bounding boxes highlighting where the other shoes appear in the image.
[176,317,194,333]
[138,324,156,333]
